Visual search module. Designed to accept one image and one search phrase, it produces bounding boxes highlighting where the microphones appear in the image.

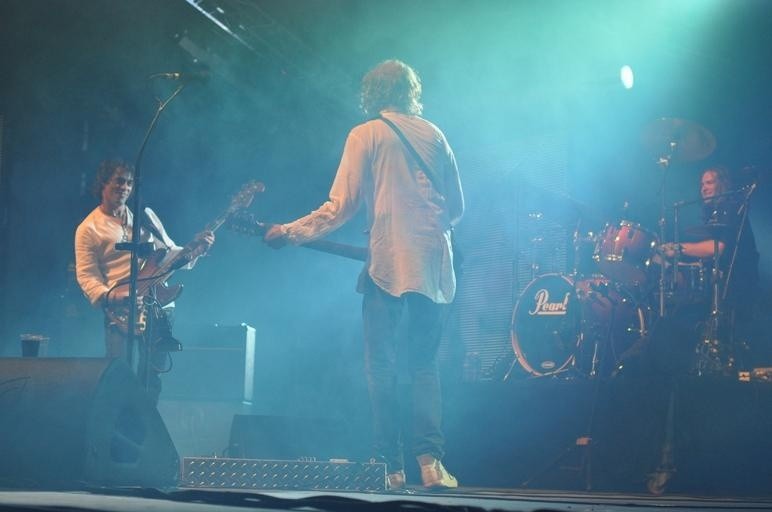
[160,70,214,81]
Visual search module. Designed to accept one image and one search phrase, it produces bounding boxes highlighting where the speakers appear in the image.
[1,355,181,489]
[193,322,257,402]
[229,413,367,461]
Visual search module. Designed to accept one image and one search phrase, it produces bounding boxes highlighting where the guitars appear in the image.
[100,178,266,340]
[224,208,465,294]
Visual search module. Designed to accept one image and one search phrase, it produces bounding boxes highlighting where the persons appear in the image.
[257,58,466,492]
[75,153,214,408]
[653,163,758,331]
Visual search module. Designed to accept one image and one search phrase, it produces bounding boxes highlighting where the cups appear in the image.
[20,335,50,358]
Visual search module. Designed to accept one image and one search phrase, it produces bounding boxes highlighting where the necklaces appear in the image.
[121,214,130,242]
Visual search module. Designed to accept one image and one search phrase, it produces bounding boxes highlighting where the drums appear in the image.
[646,260,706,301]
[591,217,659,286]
[510,272,644,380]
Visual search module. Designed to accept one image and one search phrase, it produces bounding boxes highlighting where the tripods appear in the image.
[520,280,641,493]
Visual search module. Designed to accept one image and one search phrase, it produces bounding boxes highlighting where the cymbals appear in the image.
[641,117,716,164]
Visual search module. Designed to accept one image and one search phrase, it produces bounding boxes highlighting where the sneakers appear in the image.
[387,470,407,490]
[416,454,459,490]
